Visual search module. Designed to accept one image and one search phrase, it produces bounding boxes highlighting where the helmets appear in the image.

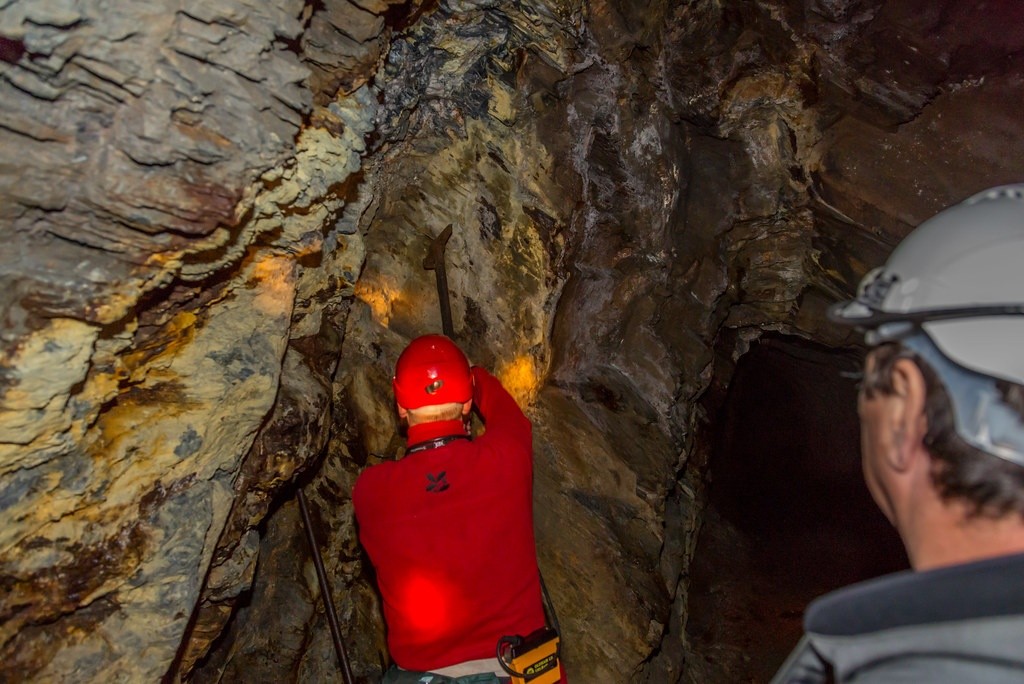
[822,183,1024,383]
[390,332,476,410]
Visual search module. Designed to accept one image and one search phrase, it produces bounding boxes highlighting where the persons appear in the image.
[349,332,569,681]
[773,181,1024,684]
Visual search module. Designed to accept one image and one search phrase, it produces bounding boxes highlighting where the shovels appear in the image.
[423,221,454,341]
